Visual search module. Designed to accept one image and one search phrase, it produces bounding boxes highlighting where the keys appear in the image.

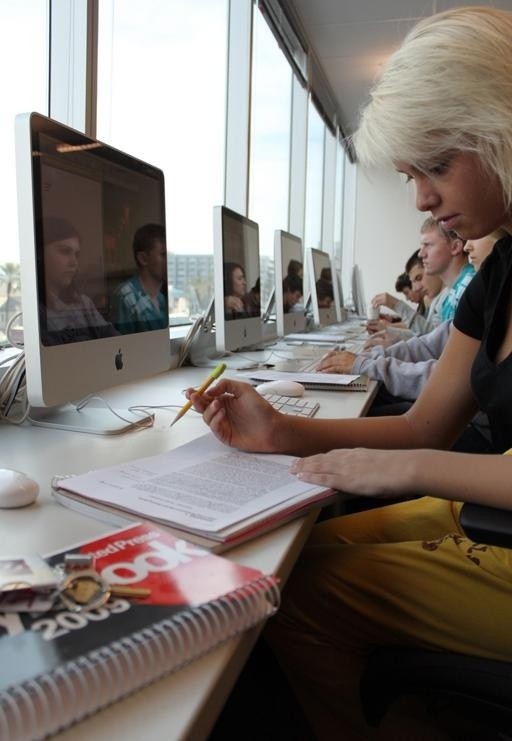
[58,569,150,612]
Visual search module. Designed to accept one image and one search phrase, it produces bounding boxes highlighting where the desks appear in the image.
[2,316,381,741]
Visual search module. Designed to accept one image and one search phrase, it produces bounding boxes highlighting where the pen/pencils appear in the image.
[169,363,226,428]
[236,364,275,371]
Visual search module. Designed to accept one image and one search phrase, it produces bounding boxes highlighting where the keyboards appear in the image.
[262,393,319,419]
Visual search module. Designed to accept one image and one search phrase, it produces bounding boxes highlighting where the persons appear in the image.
[186,6,510,741]
[222,263,260,319]
[281,259,303,313]
[44,217,111,332]
[314,214,498,452]
[111,224,168,335]
[316,268,333,308]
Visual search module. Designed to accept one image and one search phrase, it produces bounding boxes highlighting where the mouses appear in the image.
[0,467,39,509]
[256,380,305,397]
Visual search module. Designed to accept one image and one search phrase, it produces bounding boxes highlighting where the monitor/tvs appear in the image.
[14,111,171,436]
[191,205,369,371]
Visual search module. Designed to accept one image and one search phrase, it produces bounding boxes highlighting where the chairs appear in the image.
[372,499,512,741]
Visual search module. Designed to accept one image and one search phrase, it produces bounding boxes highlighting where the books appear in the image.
[249,370,369,392]
[0,519,282,741]
[49,429,340,555]
